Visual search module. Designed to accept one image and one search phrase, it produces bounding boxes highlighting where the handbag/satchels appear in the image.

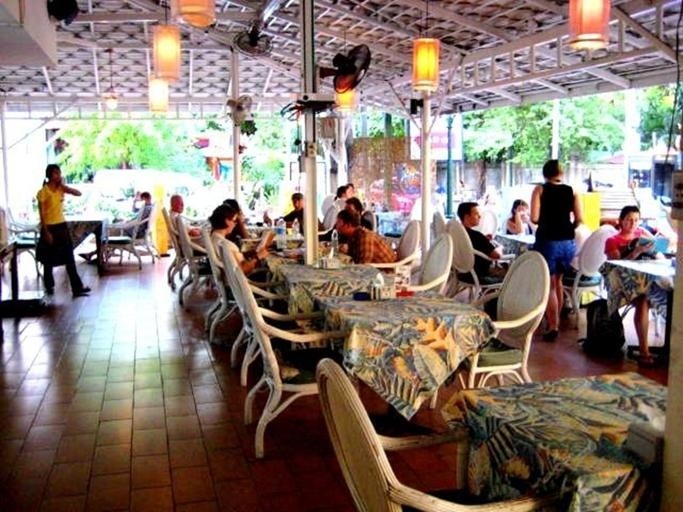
[583,300,625,362]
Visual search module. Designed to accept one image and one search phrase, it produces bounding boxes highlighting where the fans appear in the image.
[232,23,274,57]
[226,95,253,125]
[320,44,371,95]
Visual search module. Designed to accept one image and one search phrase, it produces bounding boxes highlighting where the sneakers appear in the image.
[79,253,91,260]
[543,328,558,340]
[73,286,90,294]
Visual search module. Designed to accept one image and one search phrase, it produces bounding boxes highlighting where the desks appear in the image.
[36,213,109,280]
[498,232,538,257]
[318,298,497,423]
[271,260,398,330]
[600,254,679,360]
[440,370,669,511]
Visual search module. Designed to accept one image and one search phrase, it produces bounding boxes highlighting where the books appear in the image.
[638,231,670,256]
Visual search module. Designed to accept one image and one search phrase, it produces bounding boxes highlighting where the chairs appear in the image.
[444,217,515,314]
[560,224,622,330]
[367,220,423,286]
[104,200,156,271]
[158,206,355,461]
[388,230,453,299]
[465,250,549,389]
[1,206,45,280]
[433,211,454,296]
[314,354,540,511]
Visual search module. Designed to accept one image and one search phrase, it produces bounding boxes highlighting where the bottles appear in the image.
[331,228,339,257]
[276,217,300,249]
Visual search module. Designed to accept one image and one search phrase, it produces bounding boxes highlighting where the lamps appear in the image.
[148,0,216,113]
[104,47,118,103]
[334,20,355,108]
[569,0,611,51]
[412,0,440,93]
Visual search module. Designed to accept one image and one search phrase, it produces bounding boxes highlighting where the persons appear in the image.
[529,160,582,341]
[507,159,508,160]
[455,201,507,288]
[78,191,152,263]
[562,212,599,284]
[504,199,534,235]
[36,163,91,296]
[604,205,663,365]
[170,183,399,322]
